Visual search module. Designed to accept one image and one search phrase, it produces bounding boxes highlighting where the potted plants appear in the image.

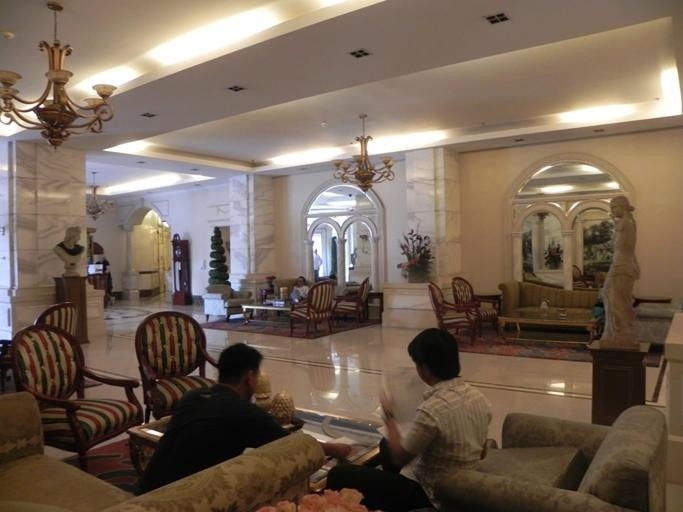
[522,231,534,273]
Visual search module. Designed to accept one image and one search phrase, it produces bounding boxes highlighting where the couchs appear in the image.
[202,285,255,324]
[631,298,683,354]
[21,391,326,512]
[434,403,668,511]
[497,280,602,336]
[272,278,313,302]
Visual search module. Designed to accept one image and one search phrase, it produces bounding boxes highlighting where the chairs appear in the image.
[332,281,370,324]
[451,276,501,338]
[135,310,222,426]
[290,280,335,337]
[0,302,79,392]
[337,276,370,299]
[35,323,145,482]
[427,282,482,346]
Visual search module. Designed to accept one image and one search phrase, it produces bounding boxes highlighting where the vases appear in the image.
[549,262,559,270]
[407,267,426,284]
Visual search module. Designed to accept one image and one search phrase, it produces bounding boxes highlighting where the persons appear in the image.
[327,328,494,511]
[313,248,323,282]
[133,342,351,498]
[288,276,310,303]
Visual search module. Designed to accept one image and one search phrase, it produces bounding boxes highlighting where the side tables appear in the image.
[368,291,384,324]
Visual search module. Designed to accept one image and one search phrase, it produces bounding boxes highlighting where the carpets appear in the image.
[454,327,663,365]
[200,320,370,338]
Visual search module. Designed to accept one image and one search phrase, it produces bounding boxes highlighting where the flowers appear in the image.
[397,229,435,276]
[545,241,564,265]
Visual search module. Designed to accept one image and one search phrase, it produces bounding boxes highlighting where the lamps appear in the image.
[0,0,117,150]
[85,186,115,221]
[333,113,395,192]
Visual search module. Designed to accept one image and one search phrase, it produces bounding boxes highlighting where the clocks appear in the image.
[171,233,193,306]
